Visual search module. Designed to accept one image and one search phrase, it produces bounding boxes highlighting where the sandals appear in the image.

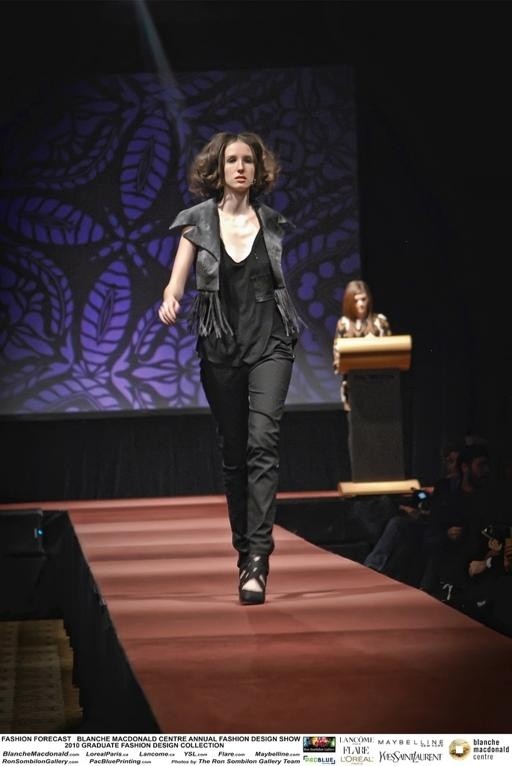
[237,555,269,606]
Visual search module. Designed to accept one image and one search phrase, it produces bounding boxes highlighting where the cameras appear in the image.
[410,487,429,502]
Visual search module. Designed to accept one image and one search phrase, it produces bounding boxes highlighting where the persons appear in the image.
[357,430,512,640]
[332,278,392,414]
[155,126,307,607]
